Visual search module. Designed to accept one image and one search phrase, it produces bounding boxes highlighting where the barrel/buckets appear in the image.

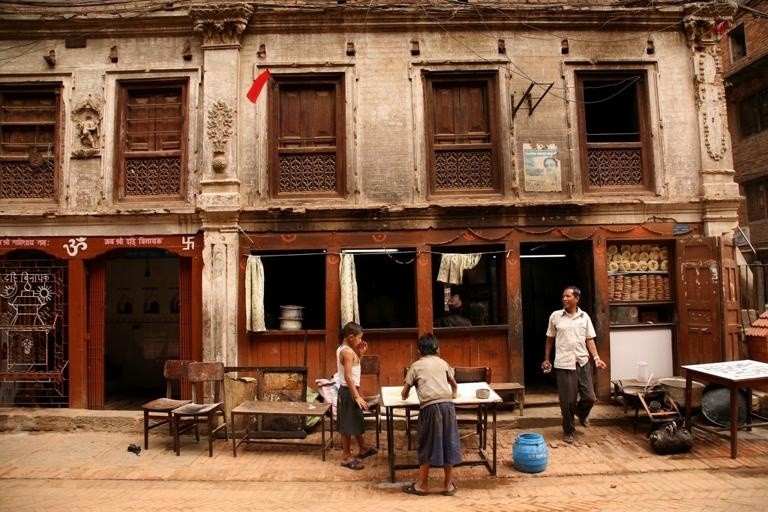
[512,432,548,473]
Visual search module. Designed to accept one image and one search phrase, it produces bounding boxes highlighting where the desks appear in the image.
[231,401,333,461]
[680,360,768,459]
[381,382,503,483]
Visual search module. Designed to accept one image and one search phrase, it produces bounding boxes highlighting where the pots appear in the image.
[702,383,746,425]
[661,378,704,408]
[620,386,664,407]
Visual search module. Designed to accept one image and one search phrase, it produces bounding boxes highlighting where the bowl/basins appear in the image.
[476,389,490,398]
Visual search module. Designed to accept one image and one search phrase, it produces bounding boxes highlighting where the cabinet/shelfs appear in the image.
[608,240,676,328]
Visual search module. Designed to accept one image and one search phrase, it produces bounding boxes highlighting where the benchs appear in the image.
[404,366,491,451]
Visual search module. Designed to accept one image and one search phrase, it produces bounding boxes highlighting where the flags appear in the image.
[245,67,272,103]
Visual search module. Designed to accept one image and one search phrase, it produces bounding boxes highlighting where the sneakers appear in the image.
[579,416,590,427]
[563,433,574,444]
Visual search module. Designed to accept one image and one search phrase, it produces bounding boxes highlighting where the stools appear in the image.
[491,383,524,416]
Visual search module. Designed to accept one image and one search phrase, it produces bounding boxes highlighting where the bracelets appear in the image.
[594,356,600,360]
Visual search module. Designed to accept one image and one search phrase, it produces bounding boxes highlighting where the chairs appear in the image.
[360,355,380,449]
[170,362,228,457]
[141,360,199,452]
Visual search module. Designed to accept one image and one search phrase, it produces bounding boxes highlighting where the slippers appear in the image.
[341,459,364,470]
[359,447,378,459]
[442,482,459,496]
[402,483,428,495]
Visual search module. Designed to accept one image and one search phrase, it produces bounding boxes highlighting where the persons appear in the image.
[79,115,100,148]
[543,157,559,185]
[335,322,380,469]
[541,285,607,443]
[434,295,472,326]
[401,331,460,497]
